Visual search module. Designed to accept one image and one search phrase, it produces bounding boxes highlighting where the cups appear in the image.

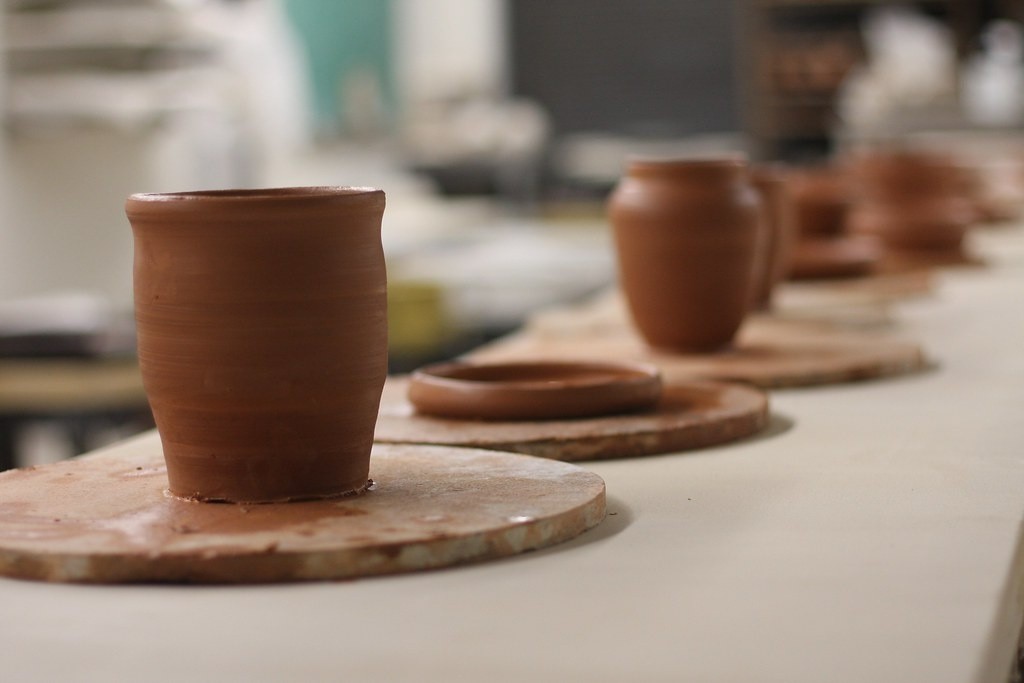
[606,158,760,353]
[124,185,388,503]
